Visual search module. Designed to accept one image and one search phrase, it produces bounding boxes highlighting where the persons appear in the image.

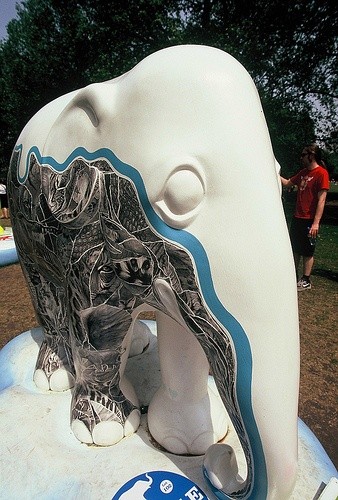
[0,180,9,219]
[281,144,330,292]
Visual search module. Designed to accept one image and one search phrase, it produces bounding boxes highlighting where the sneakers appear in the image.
[297,279,312,291]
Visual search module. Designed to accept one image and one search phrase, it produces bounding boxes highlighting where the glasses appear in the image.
[301,152,310,158]
